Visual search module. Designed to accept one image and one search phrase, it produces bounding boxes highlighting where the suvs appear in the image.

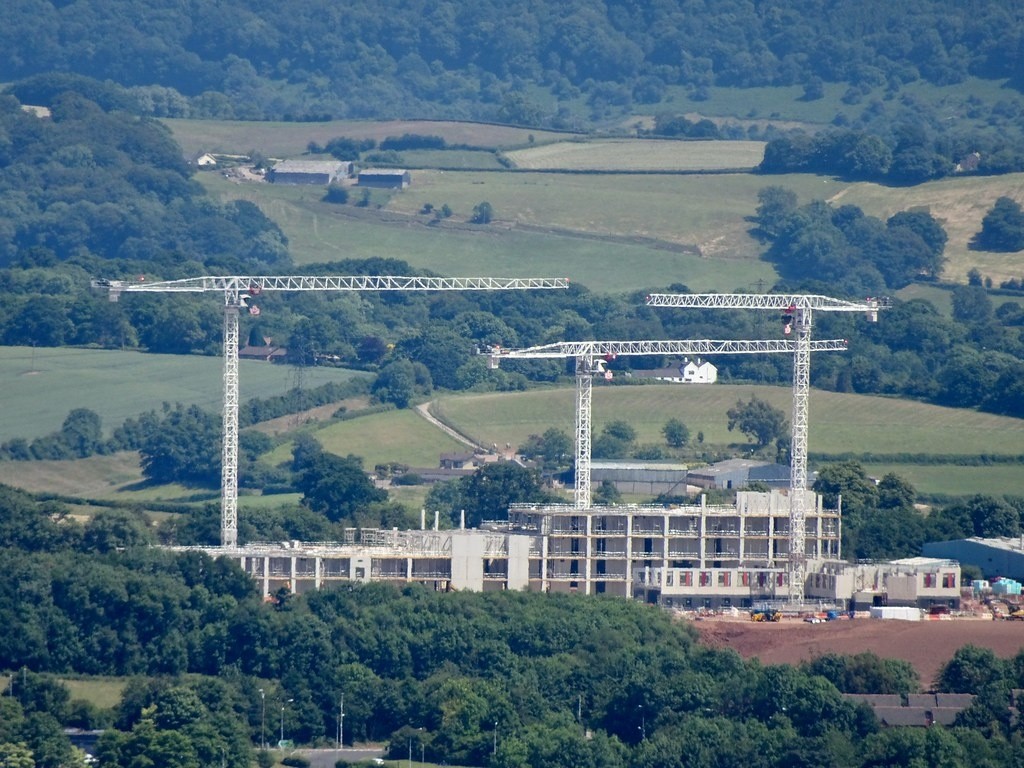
[925,605,950,619]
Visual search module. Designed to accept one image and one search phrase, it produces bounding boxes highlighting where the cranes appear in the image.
[88,273,567,547]
[480,337,848,510]
[646,292,894,601]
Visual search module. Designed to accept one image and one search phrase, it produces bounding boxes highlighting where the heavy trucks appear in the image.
[803,610,840,624]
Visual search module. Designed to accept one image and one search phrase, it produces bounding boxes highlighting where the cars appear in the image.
[372,759,384,766]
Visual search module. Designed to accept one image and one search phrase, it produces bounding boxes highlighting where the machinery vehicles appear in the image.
[752,609,781,622]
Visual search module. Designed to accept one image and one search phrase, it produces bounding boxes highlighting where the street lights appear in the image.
[409,727,421,768]
[9,675,13,694]
[259,689,265,750]
[337,715,345,753]
[281,698,294,738]
[784,708,790,718]
[494,722,498,756]
[638,705,645,741]
